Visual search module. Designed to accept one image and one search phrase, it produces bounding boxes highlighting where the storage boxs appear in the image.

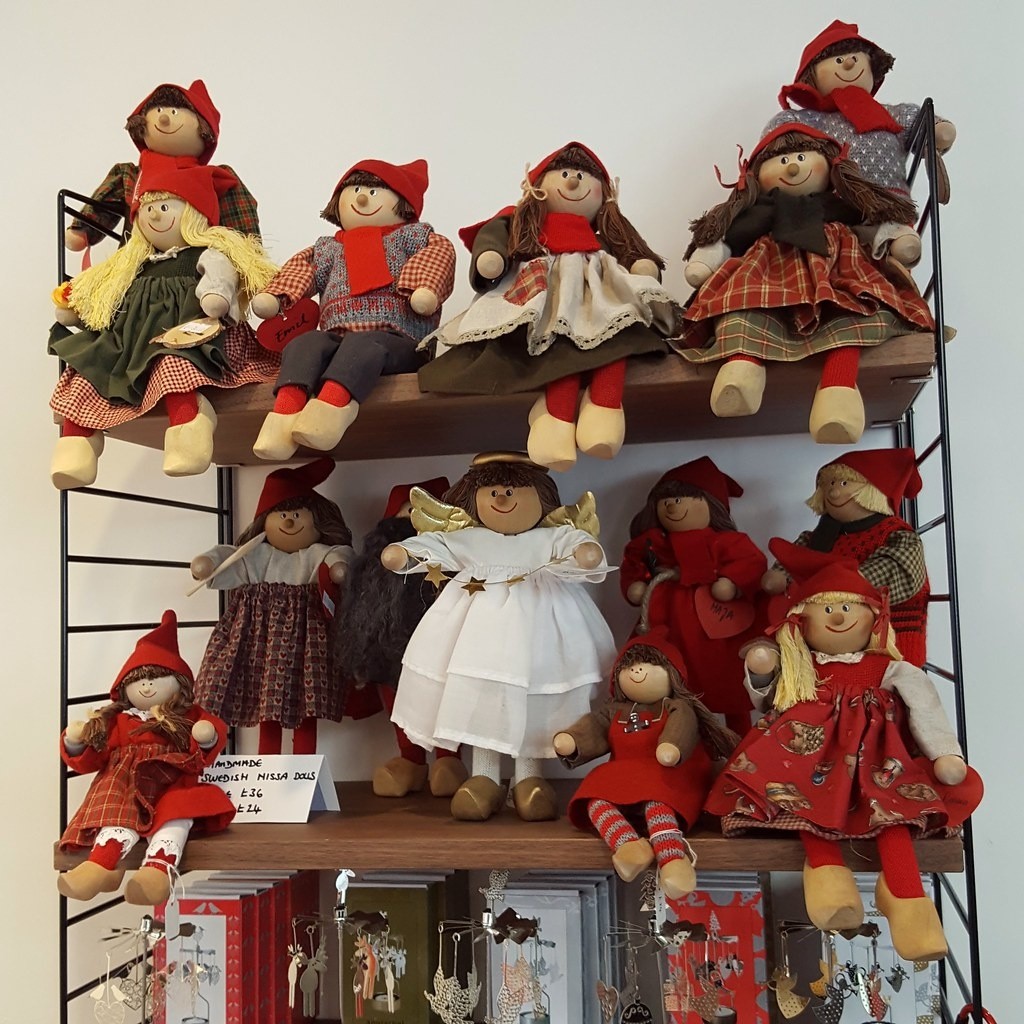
[654,870,772,1024]
[339,870,474,1024]
[821,872,942,1024]
[487,870,627,1023]
[154,870,319,1024]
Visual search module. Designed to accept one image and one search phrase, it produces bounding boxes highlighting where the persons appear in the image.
[419,142,675,470]
[701,536,985,962]
[55,447,930,905]
[555,627,740,897]
[53,18,953,487]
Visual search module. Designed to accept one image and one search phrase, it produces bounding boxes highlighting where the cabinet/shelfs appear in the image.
[56,97,982,1024]
